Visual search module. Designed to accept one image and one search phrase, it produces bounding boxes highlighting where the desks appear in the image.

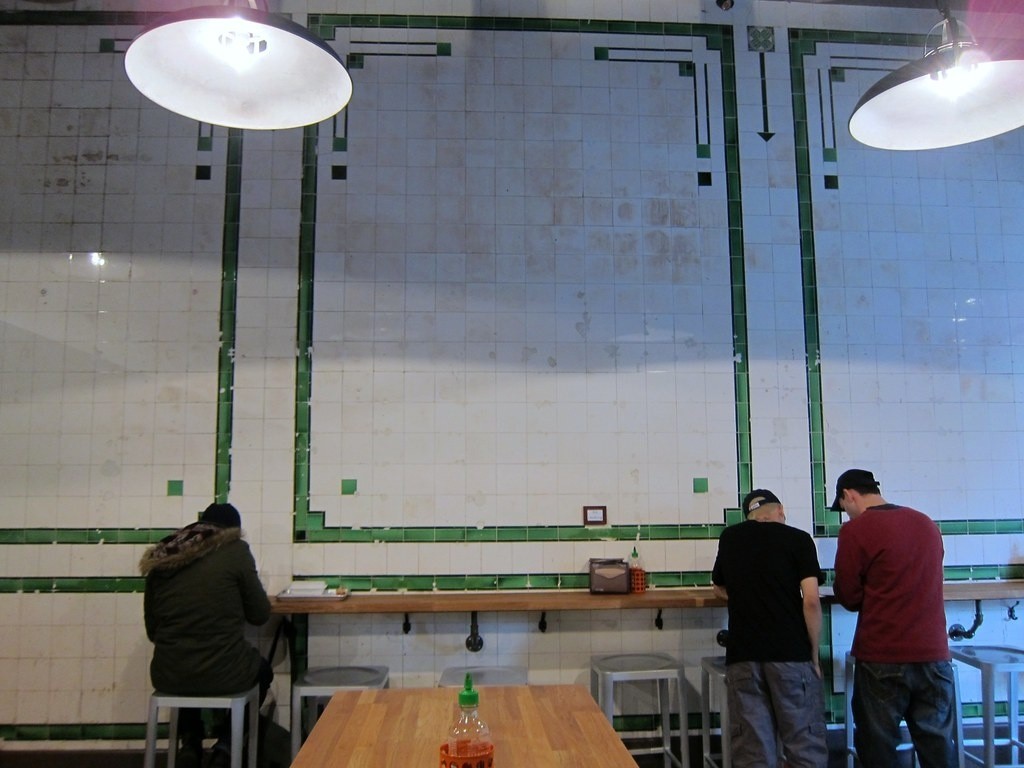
[290,684,640,768]
[268,577,1024,652]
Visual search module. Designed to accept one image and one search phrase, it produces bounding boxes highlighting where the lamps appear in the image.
[848,1,1023,151]
[124,0,354,130]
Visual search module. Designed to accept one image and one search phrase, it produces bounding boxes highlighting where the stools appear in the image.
[438,666,527,687]
[143,683,259,768]
[590,652,690,768]
[844,652,966,768]
[290,666,390,762]
[946,646,1024,768]
[700,657,787,768]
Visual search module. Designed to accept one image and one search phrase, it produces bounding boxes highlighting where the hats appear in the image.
[201,502,241,529]
[742,488,780,518]
[830,469,880,511]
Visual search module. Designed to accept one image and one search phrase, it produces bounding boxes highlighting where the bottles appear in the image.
[450,672,490,754]
[630,547,641,571]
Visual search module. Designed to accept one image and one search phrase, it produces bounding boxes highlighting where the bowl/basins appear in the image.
[288,581,328,594]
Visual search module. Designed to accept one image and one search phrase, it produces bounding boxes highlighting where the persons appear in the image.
[831,469,959,768]
[712,488,829,768]
[140,503,274,768]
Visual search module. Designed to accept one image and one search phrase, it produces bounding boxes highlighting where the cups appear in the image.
[440,742,495,767]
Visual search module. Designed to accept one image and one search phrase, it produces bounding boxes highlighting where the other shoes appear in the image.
[210,741,230,768]
[176,744,201,768]
